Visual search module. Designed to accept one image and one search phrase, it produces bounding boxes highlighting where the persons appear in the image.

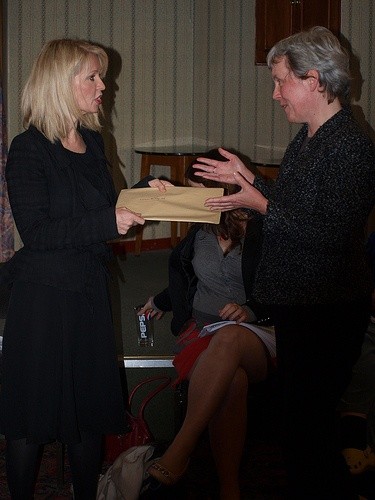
[0,39,177,500]
[138,146,269,500]
[192,24,374,500]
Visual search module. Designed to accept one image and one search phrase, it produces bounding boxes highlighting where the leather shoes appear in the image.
[147,455,194,484]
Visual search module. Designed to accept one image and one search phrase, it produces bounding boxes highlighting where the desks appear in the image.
[135,144,213,256]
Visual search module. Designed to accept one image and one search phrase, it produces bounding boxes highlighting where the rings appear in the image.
[212,166,216,173]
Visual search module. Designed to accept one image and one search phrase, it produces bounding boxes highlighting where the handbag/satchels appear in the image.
[102,375,172,467]
[96,444,154,500]
